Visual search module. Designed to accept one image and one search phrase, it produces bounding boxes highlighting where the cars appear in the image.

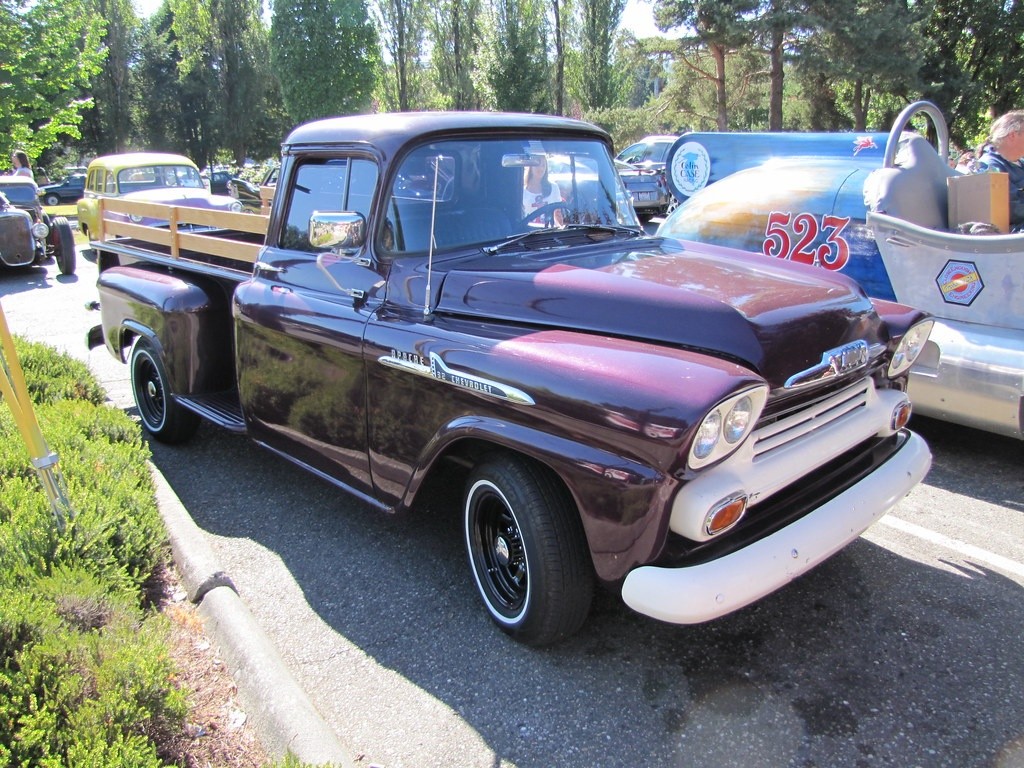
[76,152,246,254]
[647,97,1024,449]
[63,166,90,178]
[37,173,86,207]
[0,174,77,275]
[109,134,685,228]
[32,167,51,187]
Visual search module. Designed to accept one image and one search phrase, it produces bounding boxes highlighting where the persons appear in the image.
[970,109,1024,224]
[524,155,563,226]
[4,151,33,177]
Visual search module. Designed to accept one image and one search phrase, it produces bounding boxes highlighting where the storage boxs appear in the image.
[947,170,1010,235]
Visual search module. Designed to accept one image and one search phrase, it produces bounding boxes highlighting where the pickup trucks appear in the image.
[82,109,936,651]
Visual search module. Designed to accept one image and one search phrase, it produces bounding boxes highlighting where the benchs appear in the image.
[399,205,513,251]
